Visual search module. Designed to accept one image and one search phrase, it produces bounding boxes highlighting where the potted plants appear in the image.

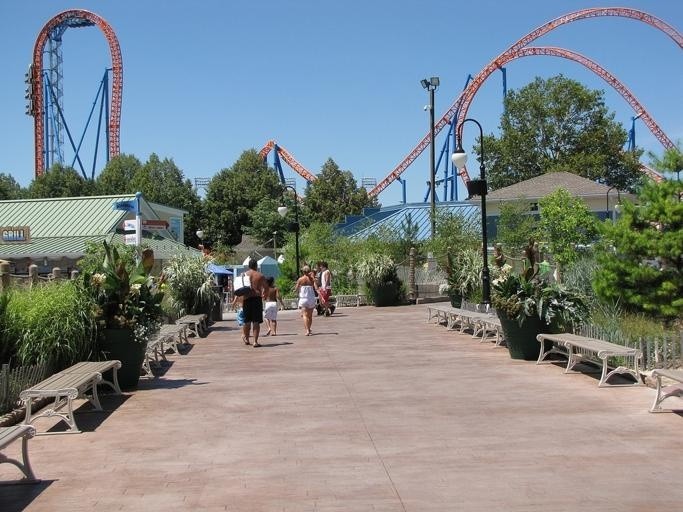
[369,264,407,307]
[162,246,215,328]
[438,245,472,308]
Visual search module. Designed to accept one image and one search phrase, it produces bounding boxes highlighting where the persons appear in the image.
[291,261,336,336]
[230,295,243,326]
[238,259,268,348]
[262,276,285,336]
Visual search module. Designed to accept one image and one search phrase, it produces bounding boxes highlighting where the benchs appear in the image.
[143,313,208,375]
[647,369,683,412]
[19,360,122,434]
[0,425,42,484]
[425,306,505,349]
[533,332,644,387]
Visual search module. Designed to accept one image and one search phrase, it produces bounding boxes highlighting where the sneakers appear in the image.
[265,328,271,336]
[331,306,335,314]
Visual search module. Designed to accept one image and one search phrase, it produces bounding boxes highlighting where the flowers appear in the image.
[77,237,168,346]
[489,256,594,327]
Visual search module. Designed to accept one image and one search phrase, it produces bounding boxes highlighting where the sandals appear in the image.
[241,335,250,345]
[253,342,261,346]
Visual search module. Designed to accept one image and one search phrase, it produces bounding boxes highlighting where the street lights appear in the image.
[606,185,624,219]
[631,112,643,151]
[278,186,299,279]
[452,118,491,312]
[197,217,211,252]
[420,76,440,242]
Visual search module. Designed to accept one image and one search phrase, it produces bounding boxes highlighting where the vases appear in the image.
[98,338,146,387]
[500,318,554,360]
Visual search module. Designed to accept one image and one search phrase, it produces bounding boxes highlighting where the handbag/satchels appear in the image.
[232,273,252,297]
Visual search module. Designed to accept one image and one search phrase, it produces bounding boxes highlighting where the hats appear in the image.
[301,266,311,273]
